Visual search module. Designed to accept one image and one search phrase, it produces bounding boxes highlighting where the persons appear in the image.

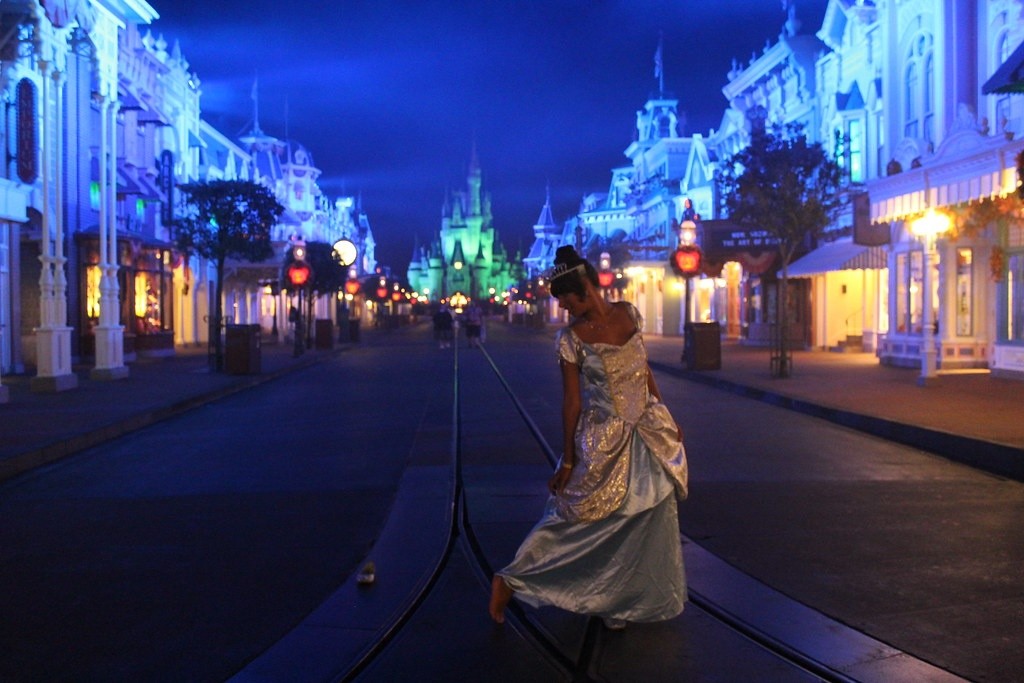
[431,301,482,348]
[490,246,688,629]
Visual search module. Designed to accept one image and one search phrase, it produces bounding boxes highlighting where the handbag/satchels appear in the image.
[481,327,486,343]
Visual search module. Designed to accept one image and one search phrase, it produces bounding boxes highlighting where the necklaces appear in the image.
[582,313,613,330]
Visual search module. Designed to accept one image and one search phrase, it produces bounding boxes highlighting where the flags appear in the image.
[654,48,662,81]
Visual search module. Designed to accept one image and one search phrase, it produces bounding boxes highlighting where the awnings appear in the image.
[778,239,889,277]
[78,225,171,250]
[695,220,793,254]
[981,40,1024,96]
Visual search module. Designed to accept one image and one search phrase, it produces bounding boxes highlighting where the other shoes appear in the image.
[355,559,375,584]
[604,616,626,629]
